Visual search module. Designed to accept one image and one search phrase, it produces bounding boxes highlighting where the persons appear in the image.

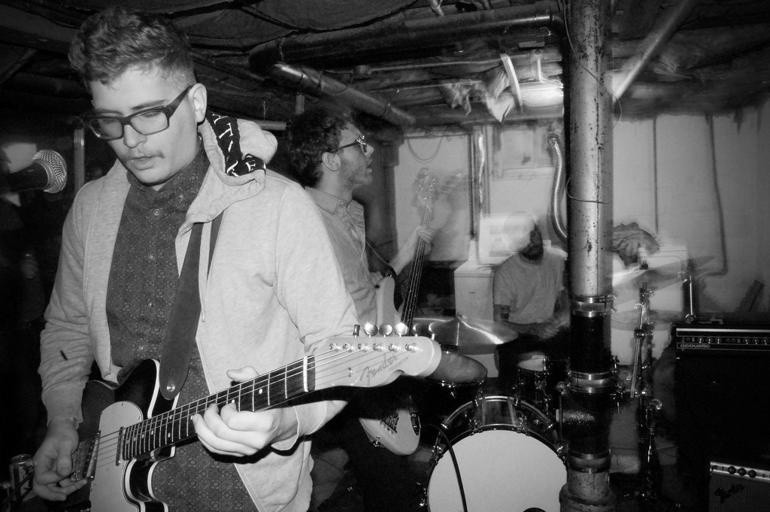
[29,7,366,511]
[285,104,439,510]
[494,211,571,373]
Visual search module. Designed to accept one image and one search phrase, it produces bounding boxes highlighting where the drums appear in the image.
[417,390,568,512]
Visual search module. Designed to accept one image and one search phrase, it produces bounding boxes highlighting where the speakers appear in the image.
[675,313,770,483]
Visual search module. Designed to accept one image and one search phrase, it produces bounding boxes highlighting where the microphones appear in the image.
[0,148,69,194]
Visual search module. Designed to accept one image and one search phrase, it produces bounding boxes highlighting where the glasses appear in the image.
[327,133,370,159]
[78,84,192,142]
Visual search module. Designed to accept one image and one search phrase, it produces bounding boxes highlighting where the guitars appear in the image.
[30,323,441,512]
[349,170,439,454]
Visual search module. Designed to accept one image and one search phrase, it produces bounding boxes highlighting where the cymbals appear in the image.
[411,315,516,348]
[613,254,711,303]
